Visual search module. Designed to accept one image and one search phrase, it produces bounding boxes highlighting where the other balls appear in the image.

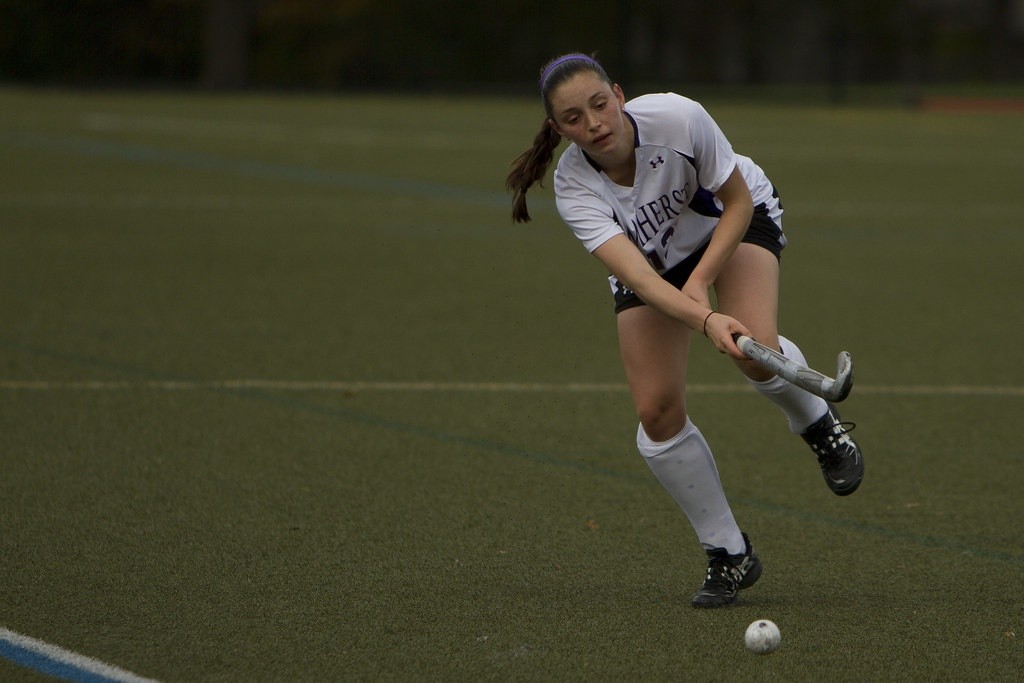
[744,618,782,656]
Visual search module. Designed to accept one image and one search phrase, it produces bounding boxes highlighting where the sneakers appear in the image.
[691,531,762,609]
[799,399,865,497]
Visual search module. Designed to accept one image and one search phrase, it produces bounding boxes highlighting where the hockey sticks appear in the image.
[730,330,856,403]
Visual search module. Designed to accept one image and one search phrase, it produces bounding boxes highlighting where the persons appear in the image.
[507,52,865,606]
